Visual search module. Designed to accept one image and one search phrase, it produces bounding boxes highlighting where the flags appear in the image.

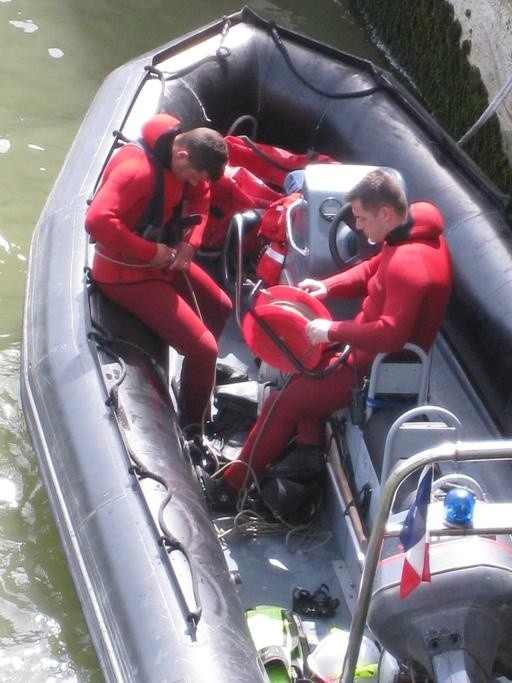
[398,463,432,601]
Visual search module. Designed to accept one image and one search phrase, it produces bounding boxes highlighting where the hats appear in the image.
[388,199,444,242]
[141,113,182,153]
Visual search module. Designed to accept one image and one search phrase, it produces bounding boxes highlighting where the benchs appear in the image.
[345,342,466,537]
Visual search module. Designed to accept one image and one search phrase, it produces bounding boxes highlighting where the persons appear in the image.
[193,168,452,513]
[85,113,234,473]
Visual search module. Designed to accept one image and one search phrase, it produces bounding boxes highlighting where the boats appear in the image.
[19,4,512,683]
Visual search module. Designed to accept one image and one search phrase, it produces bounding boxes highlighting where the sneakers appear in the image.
[264,443,325,479]
[200,474,239,515]
